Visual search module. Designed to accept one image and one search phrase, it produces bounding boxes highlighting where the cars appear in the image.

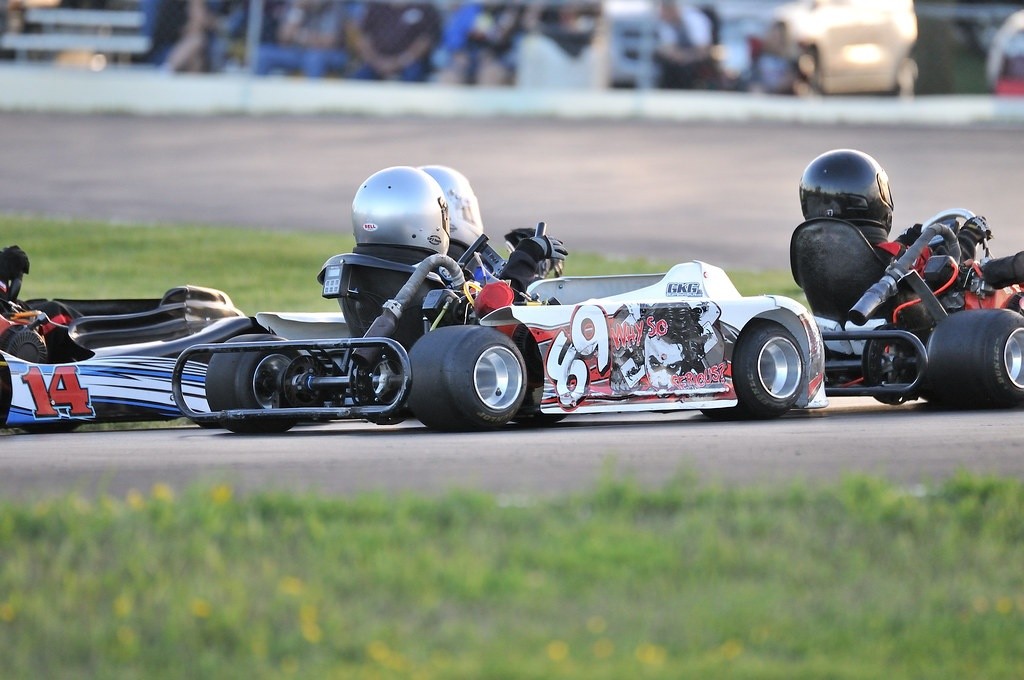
[0,0,1024,94]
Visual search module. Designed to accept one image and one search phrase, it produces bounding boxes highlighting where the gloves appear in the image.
[0,245,30,281]
[530,234,568,260]
[504,228,537,252]
[898,223,923,246]
[959,214,992,243]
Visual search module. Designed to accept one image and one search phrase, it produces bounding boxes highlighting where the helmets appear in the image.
[418,164,484,246]
[799,147,893,234]
[351,166,450,256]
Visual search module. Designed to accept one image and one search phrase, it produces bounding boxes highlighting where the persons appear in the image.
[797,148,993,287]
[349,163,571,304]
[132,0,728,90]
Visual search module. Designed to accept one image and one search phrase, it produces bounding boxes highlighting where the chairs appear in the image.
[316,252,449,355]
[790,216,898,362]
